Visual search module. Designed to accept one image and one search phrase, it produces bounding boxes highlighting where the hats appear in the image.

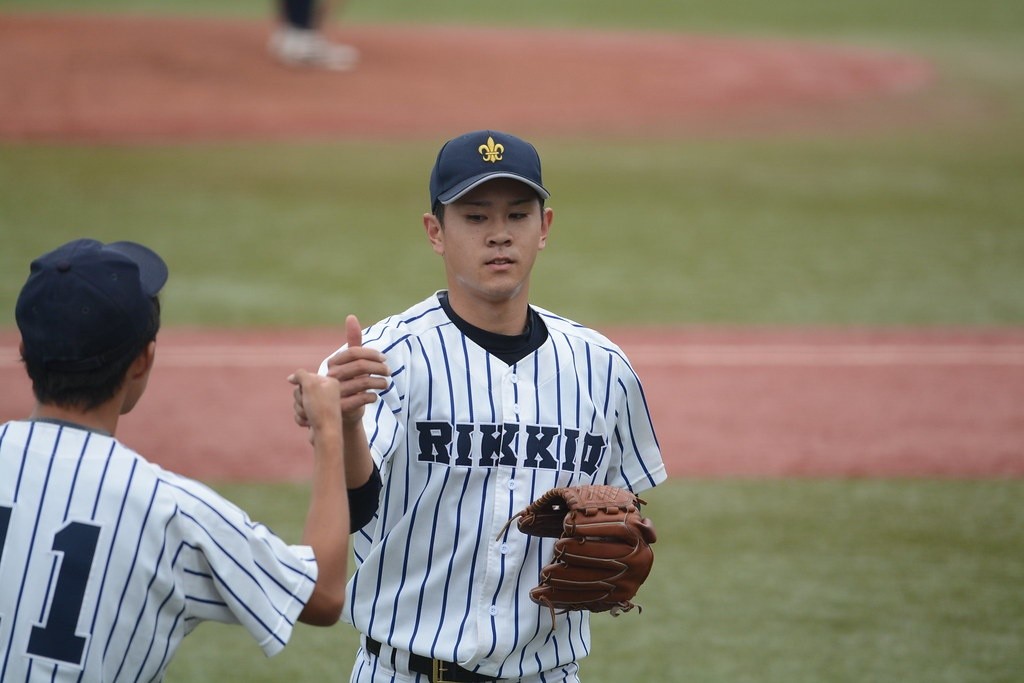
[15,238,168,362]
[429,130,549,213]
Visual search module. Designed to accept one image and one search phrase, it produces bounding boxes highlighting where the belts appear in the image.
[365,636,500,683]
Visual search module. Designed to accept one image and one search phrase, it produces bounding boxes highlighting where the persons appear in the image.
[0,129,350,683]
[317,130,669,683]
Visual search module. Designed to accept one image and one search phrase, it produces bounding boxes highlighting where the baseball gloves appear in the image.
[496,484,658,630]
